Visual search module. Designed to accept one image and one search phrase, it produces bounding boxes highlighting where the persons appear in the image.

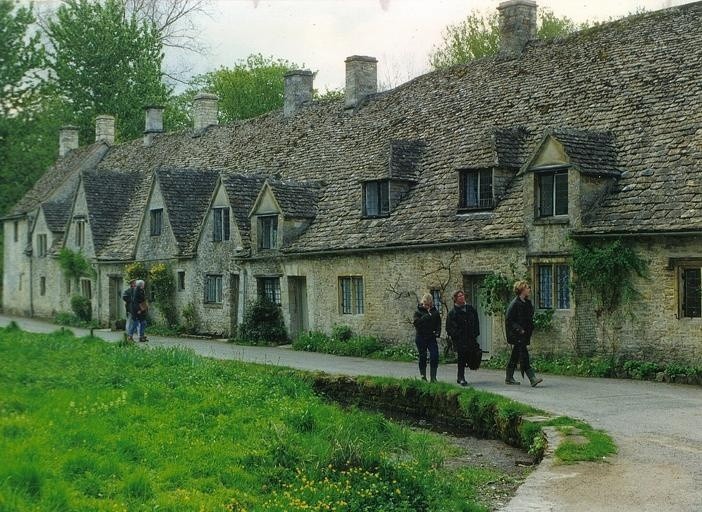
[127,279,149,343]
[122,279,147,338]
[445,290,480,385]
[504,280,543,387]
[413,293,442,383]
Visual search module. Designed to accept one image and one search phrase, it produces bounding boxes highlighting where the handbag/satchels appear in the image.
[139,302,148,312]
[464,339,482,370]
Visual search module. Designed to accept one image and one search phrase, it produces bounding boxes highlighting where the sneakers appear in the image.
[128,336,149,342]
[457,376,467,385]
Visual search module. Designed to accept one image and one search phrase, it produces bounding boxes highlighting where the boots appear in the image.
[505,367,520,385]
[525,367,542,387]
